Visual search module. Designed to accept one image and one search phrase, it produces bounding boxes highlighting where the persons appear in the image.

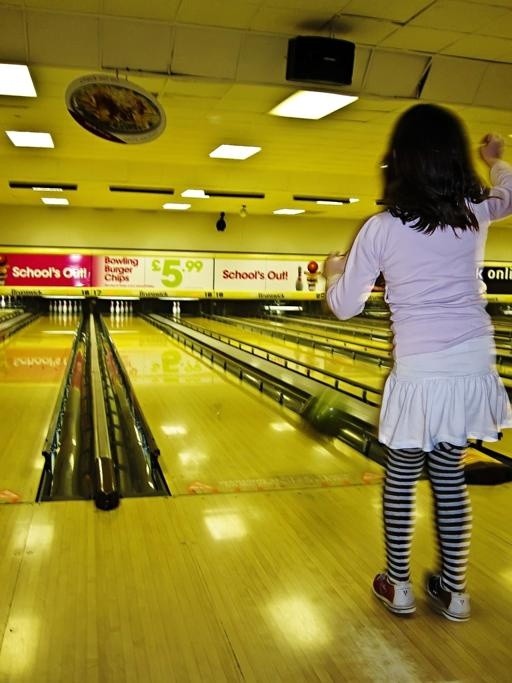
[320,99,512,624]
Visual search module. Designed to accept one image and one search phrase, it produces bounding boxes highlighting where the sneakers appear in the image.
[372,573,417,614]
[427,574,471,622]
